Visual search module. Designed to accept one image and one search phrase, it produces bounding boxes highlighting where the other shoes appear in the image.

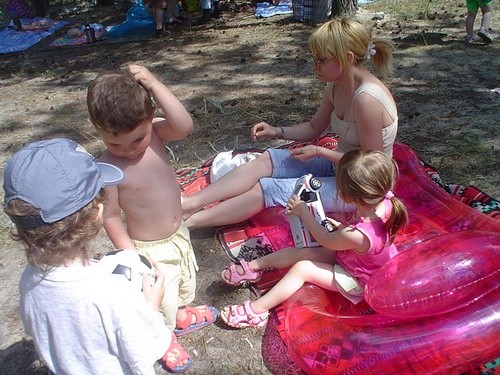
[155,23,172,38]
[169,17,184,24]
[212,3,221,19]
[198,9,213,21]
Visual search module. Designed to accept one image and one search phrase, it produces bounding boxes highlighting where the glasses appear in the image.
[313,57,329,66]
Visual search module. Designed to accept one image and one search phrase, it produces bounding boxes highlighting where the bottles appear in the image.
[84,24,96,43]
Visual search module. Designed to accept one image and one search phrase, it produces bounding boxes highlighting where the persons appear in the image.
[180,17,398,232]
[155,0,183,38]
[219,147,409,329]
[87,64,221,373]
[0,138,172,375]
[195,0,222,22]
[464,0,493,45]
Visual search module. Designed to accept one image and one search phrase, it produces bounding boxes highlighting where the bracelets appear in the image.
[277,126,284,139]
[314,144,319,158]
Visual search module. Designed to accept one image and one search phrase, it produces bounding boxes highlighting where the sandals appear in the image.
[173,304,222,337]
[220,300,272,328]
[161,332,192,373]
[220,258,265,287]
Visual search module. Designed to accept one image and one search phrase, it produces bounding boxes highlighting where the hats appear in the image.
[3,137,127,228]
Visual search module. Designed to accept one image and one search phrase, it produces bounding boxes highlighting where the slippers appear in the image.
[477,31,494,43]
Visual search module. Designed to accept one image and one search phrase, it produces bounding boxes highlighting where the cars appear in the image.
[285,172,333,248]
